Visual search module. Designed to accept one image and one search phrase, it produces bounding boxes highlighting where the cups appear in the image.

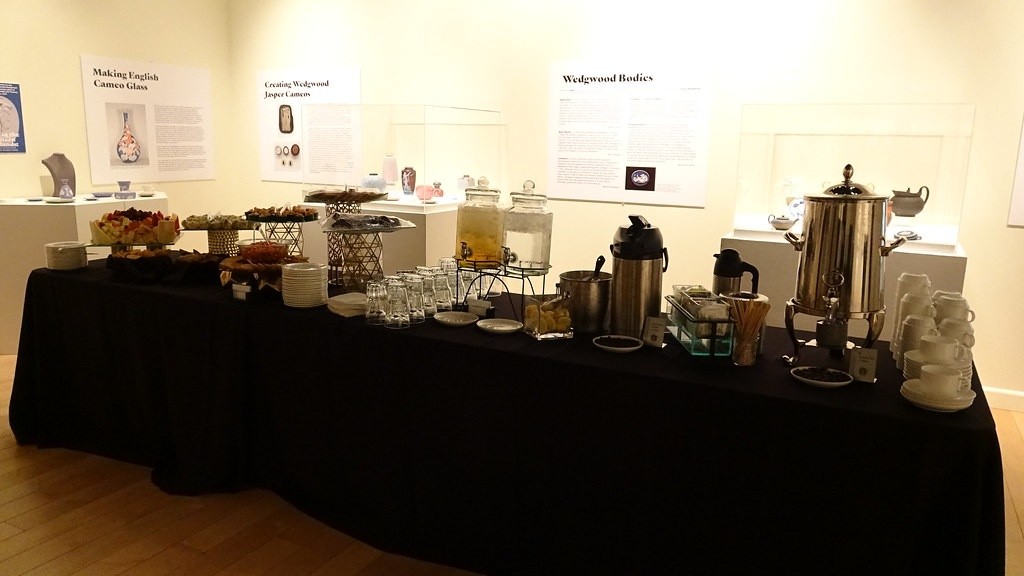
[365,257,503,330]
[733,333,759,366]
[889,271,975,398]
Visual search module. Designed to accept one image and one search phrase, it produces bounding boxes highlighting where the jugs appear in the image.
[610,213,668,338]
[712,248,759,296]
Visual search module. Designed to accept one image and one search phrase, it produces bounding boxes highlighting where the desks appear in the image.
[9,249,1006,576]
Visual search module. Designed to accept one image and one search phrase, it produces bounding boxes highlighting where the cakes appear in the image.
[90,208,179,245]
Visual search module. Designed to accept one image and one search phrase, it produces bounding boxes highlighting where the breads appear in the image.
[525,304,572,334]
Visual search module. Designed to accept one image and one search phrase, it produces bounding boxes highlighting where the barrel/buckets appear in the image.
[556,271,612,337]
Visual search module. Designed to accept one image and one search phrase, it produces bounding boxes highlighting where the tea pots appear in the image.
[768,213,799,231]
[890,186,930,217]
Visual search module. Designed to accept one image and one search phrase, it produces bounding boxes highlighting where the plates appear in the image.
[433,311,479,326]
[790,366,854,388]
[281,262,328,309]
[592,335,645,352]
[900,350,976,414]
[476,318,524,334]
[44,241,88,270]
